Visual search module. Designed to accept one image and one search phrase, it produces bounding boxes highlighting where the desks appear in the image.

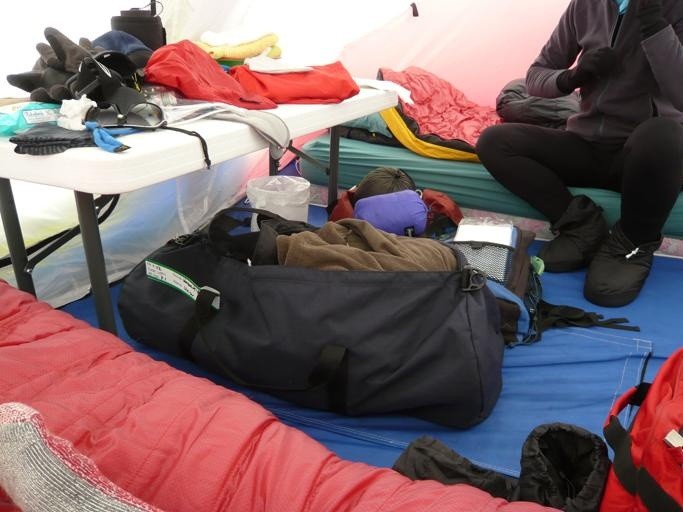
[0,84,400,338]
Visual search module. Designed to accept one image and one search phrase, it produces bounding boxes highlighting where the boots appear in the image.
[537,195,608,273]
[584,215,663,309]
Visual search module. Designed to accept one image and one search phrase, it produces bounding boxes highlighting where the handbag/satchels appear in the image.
[117,207,504,432]
[598,346,683,512]
[332,167,463,235]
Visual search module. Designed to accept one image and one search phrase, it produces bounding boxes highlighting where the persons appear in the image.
[476,1,683,307]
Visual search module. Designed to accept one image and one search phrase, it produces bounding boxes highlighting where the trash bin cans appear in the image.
[246,175,311,233]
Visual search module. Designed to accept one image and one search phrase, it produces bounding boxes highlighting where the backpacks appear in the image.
[425,218,640,349]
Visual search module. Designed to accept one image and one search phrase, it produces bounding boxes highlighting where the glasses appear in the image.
[86,103,167,130]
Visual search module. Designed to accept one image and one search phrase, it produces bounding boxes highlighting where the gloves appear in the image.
[6,27,135,102]
[636,0,668,41]
[557,47,618,94]
